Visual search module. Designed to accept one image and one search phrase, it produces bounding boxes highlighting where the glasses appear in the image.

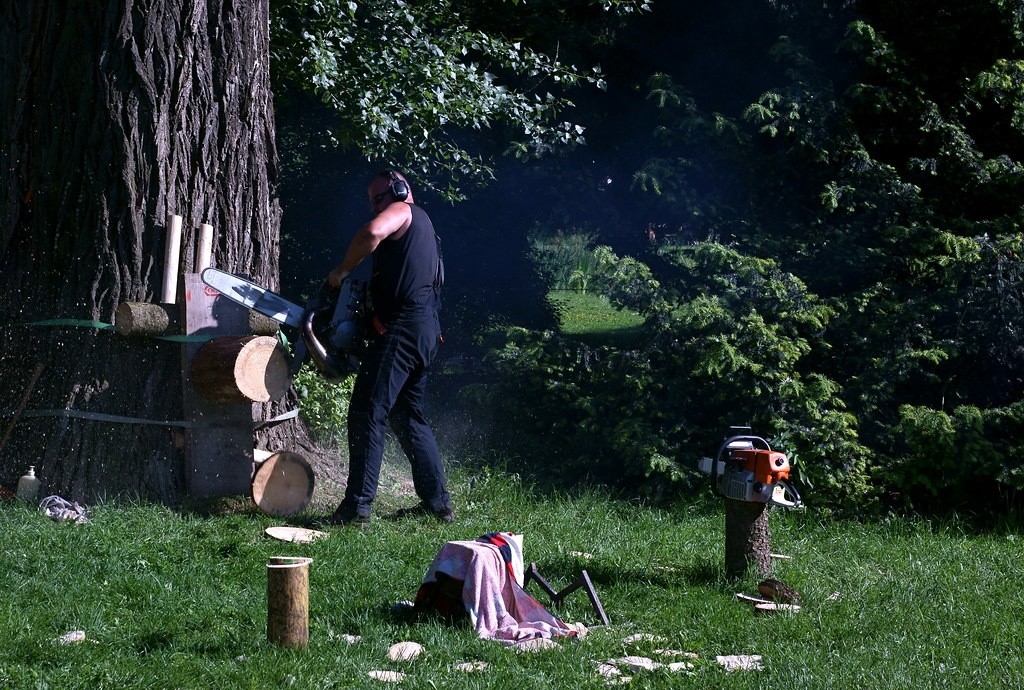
[368,190,392,206]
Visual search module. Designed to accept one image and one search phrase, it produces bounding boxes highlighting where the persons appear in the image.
[321,169,455,526]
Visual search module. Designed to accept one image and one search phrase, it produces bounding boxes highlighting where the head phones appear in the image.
[380,170,409,202]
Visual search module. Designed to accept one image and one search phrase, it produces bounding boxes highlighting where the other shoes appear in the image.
[317,505,371,525]
[385,501,453,523]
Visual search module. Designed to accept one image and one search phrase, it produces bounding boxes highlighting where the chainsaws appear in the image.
[201,255,387,379]
[698,426,802,509]
